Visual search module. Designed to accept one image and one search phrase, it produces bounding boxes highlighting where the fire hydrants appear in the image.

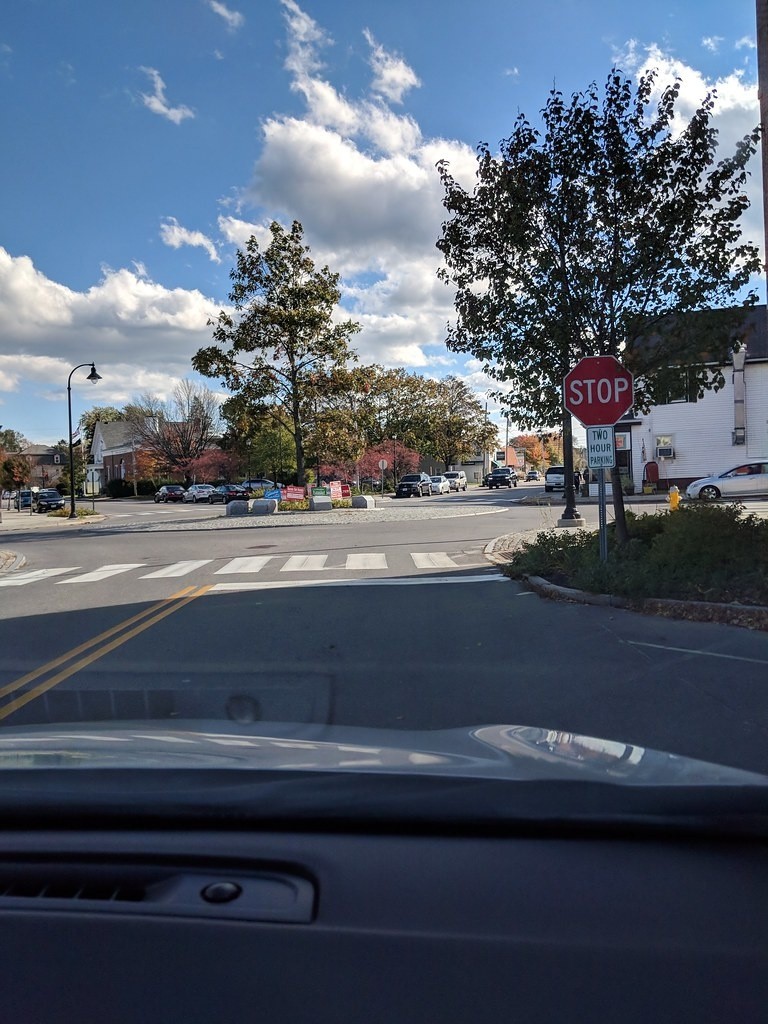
[668,484,680,512]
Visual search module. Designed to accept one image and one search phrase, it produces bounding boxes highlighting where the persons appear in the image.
[573,467,582,494]
[583,466,589,484]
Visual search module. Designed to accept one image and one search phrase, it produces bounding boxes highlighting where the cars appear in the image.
[186,484,213,503]
[432,477,452,496]
[395,474,432,496]
[486,468,516,489]
[688,455,768,502]
[38,493,64,511]
[447,472,467,490]
[152,485,184,504]
[545,467,564,490]
[242,480,283,493]
[16,491,34,509]
[208,486,250,503]
[526,471,539,481]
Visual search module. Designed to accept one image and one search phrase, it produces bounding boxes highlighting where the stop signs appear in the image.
[558,356,635,423]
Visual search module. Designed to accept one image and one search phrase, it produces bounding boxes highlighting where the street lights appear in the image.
[65,360,100,514]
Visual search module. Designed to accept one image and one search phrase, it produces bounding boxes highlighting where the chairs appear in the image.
[40,495,45,499]
[752,467,758,474]
